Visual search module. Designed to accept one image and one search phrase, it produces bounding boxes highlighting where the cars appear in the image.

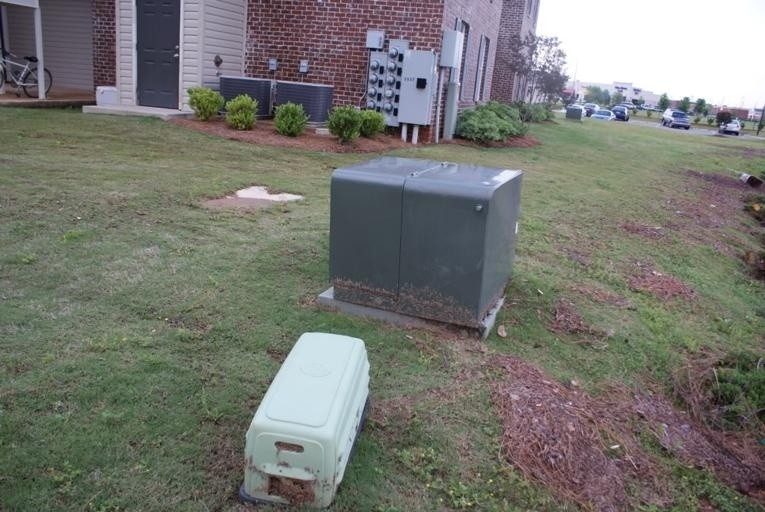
[566,102,636,121]
[720,120,740,135]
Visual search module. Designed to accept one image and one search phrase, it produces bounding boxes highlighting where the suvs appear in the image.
[662,107,689,129]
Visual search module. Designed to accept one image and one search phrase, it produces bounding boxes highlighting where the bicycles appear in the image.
[0,48,53,98]
